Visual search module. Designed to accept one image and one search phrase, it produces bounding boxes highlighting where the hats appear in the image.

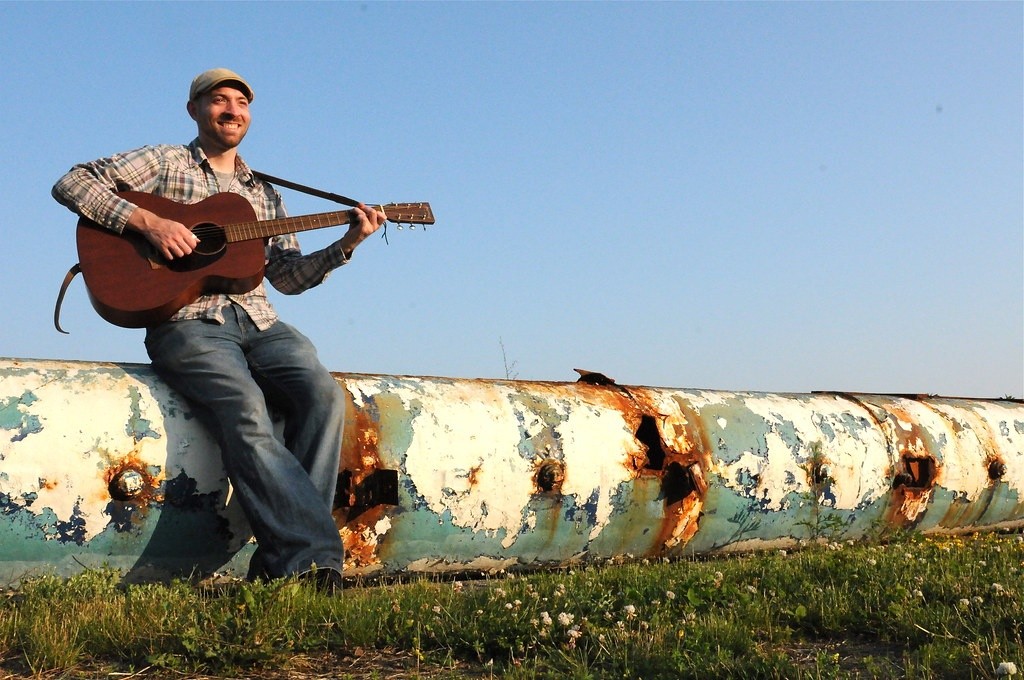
[189,69,254,103]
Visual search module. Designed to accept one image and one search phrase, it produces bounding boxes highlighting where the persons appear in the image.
[50,67,388,602]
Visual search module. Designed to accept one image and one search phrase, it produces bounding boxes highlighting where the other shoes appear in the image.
[247,571,283,584]
[308,568,342,597]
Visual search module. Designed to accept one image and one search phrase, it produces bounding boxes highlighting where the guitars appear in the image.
[76,192,435,328]
